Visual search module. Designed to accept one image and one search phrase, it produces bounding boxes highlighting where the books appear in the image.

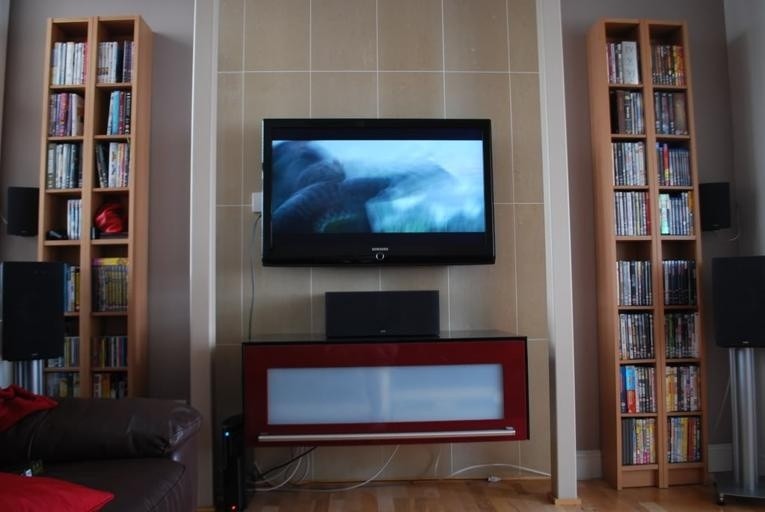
[611,142,692,186]
[620,313,699,359]
[621,418,701,465]
[615,191,694,236]
[610,91,688,135]
[42,38,134,397]
[620,365,700,414]
[616,260,697,305]
[608,42,686,86]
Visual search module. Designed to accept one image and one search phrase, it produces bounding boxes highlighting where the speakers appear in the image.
[326,291,440,342]
[7,186,38,238]
[712,257,765,348]
[0,261,67,360]
[699,181,731,231]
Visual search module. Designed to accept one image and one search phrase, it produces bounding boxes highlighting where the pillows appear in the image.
[1,471,114,510]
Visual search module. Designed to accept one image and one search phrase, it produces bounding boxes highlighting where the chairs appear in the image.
[1,382,203,511]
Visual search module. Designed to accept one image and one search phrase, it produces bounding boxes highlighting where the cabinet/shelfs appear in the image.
[584,19,711,492]
[37,16,153,402]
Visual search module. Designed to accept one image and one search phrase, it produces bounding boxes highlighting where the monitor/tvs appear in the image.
[262,119,495,265]
